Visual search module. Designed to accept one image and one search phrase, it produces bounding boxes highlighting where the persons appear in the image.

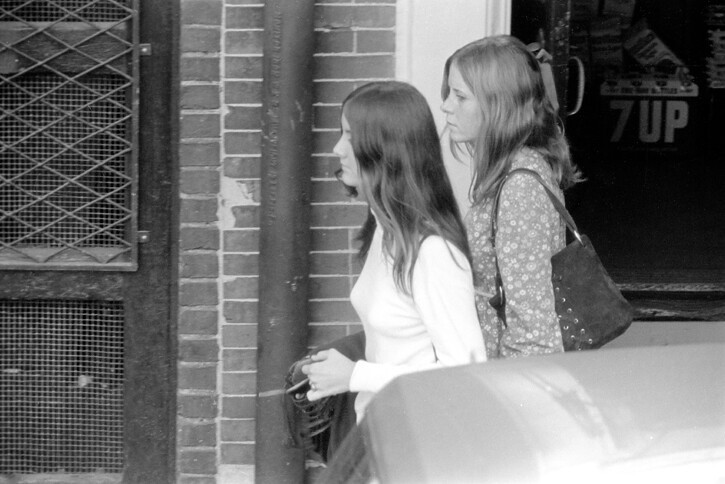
[440,34,568,360]
[302,81,487,425]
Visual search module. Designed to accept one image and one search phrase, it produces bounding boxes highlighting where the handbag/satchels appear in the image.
[488,168,636,349]
